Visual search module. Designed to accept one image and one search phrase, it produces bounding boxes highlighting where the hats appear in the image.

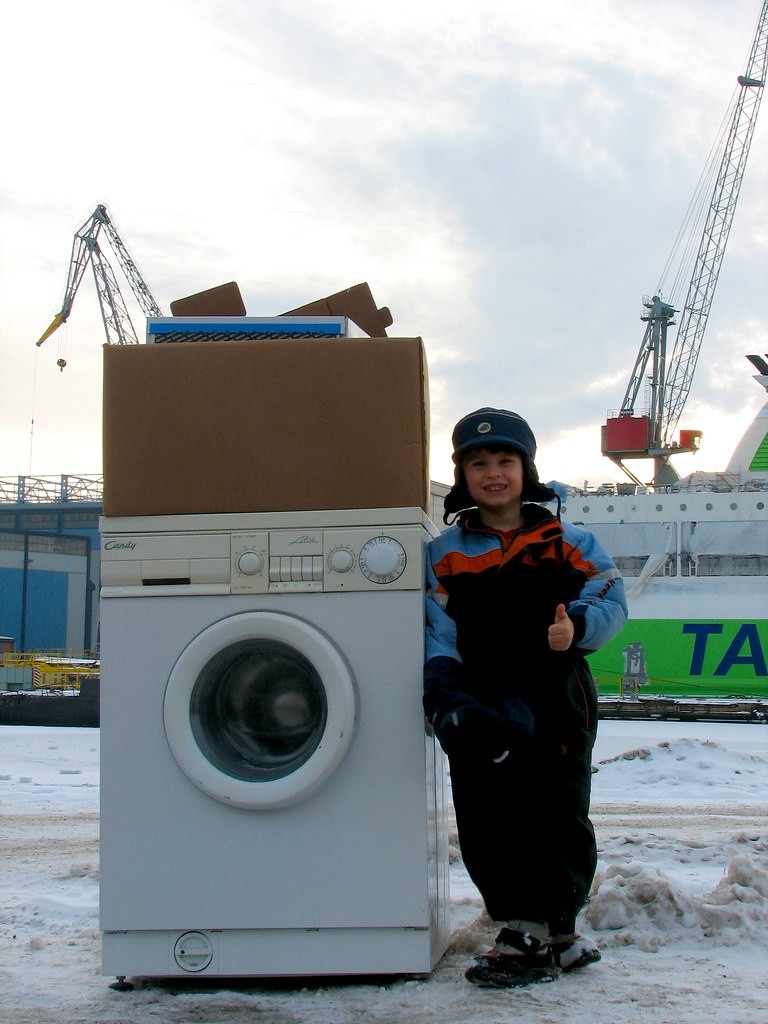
[443,406,556,513]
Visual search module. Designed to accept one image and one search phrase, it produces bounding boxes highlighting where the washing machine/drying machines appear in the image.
[99,507,450,976]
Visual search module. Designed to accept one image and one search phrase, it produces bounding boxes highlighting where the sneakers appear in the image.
[554,935,601,972]
[464,927,562,987]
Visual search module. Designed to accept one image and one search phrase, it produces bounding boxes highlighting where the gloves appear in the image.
[433,690,535,784]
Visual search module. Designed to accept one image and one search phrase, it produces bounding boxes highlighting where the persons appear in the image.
[423,407,629,988]
